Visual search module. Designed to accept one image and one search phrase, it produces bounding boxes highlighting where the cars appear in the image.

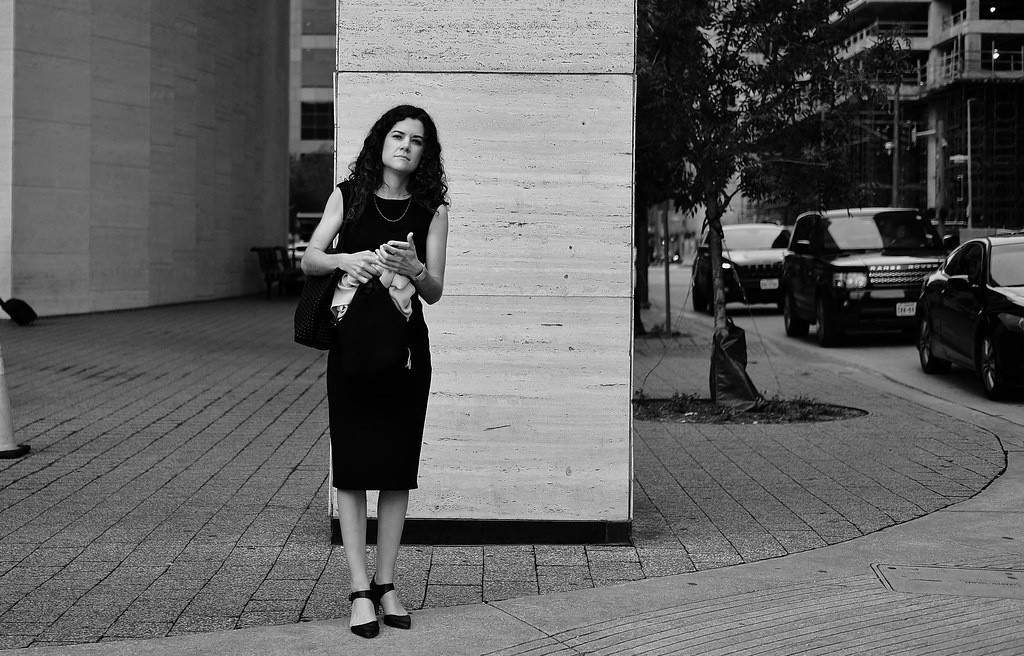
[914,232,1024,402]
[692,222,790,314]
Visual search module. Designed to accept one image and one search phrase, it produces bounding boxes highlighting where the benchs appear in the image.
[251,247,305,297]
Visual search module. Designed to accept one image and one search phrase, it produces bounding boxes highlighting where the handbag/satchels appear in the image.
[294,247,340,351]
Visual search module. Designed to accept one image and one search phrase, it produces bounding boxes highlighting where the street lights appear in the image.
[966,97,978,228]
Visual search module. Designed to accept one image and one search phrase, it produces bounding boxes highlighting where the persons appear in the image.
[301,104,452,639]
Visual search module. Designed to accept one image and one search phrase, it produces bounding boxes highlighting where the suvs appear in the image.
[777,207,961,348]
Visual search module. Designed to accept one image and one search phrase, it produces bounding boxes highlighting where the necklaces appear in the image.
[373,194,411,222]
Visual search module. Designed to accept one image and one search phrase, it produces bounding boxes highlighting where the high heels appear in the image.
[349,588,379,638]
[371,574,411,629]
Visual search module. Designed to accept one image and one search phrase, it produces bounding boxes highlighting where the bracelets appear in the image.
[413,262,427,281]
